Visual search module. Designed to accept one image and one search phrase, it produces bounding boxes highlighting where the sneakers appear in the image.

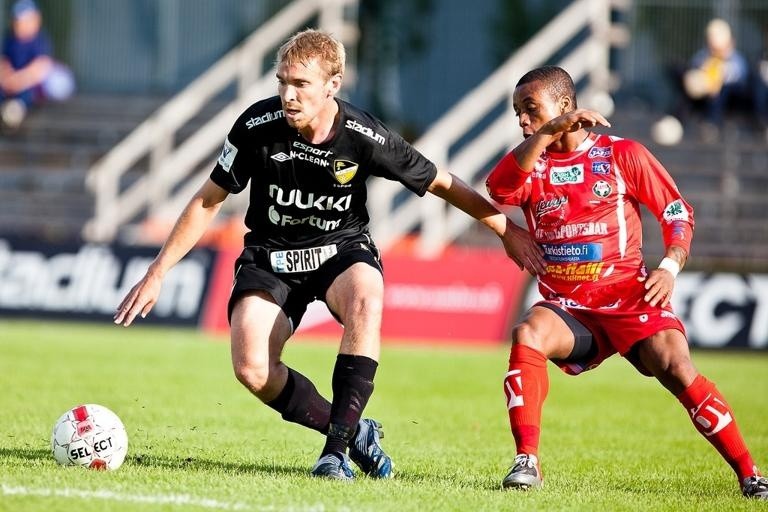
[503,453,544,489]
[742,476,768,499]
[311,451,354,482]
[349,418,395,480]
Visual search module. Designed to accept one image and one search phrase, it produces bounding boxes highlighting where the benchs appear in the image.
[0,93,238,248]
[451,89,768,269]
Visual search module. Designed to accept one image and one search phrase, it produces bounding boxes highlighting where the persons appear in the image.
[0,0,59,132]
[683,18,759,132]
[113,28,548,483]
[486,65,768,501]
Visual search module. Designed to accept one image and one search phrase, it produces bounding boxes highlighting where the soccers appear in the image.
[50,404,128,471]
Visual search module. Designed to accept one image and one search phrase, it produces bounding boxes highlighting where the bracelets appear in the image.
[657,257,680,278]
